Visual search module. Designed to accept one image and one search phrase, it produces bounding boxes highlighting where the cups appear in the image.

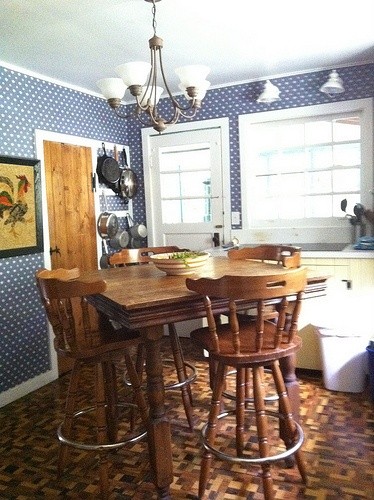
[356,224,371,241]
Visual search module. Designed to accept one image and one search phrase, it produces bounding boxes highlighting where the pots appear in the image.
[98,212,147,269]
[98,142,139,200]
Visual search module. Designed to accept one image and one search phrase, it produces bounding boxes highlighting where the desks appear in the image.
[72,254,334,500]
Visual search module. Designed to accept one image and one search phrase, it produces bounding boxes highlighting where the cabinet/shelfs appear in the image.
[219,257,374,375]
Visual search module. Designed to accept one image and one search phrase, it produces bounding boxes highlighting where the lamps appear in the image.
[256,79,281,103]
[95,0,211,135]
[318,69,345,94]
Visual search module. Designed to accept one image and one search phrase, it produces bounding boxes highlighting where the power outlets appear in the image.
[232,211,241,225]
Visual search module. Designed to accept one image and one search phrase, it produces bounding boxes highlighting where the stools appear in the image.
[35,266,149,500]
[107,246,198,432]
[210,243,302,429]
[184,265,311,500]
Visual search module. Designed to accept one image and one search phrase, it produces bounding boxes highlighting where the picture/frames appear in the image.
[0,155,45,259]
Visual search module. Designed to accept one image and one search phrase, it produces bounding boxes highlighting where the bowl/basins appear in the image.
[149,251,211,275]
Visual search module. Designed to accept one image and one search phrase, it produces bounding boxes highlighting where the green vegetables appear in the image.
[169,250,207,269]
